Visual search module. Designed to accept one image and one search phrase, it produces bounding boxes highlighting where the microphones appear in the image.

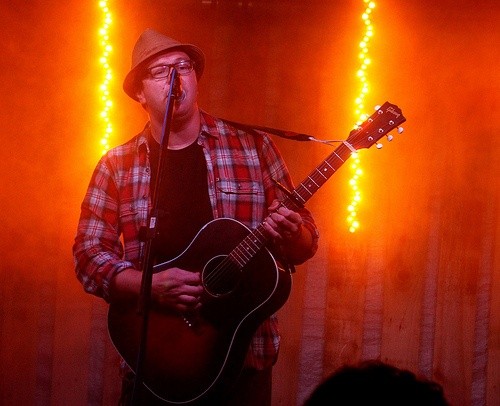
[170,69,186,103]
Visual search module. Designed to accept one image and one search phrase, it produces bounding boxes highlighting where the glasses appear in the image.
[147,60,193,79]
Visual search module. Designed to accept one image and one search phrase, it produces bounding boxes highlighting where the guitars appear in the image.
[106,101,405,406]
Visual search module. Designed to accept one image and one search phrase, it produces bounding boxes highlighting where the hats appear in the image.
[123,28,205,102]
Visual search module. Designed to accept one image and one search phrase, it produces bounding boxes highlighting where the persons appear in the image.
[72,28,320,406]
[303,359,451,406]
